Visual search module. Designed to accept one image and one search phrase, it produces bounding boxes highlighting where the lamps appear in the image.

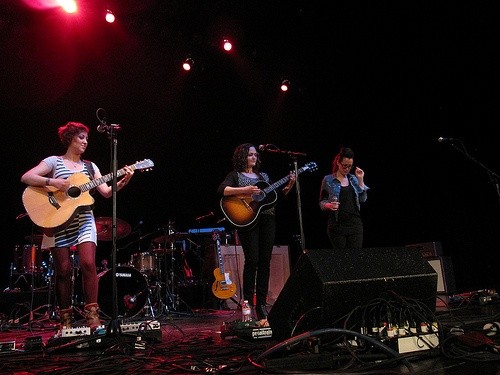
[279,79,291,92]
[105,10,115,22]
[181,57,195,71]
[220,37,233,51]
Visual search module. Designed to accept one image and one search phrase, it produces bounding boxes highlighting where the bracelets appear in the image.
[46,178,50,185]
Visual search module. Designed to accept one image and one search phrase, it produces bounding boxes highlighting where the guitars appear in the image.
[210,231,238,298]
[219,162,320,231]
[21,158,154,231]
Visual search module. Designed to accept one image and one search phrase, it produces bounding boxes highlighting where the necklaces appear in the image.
[65,155,80,168]
[21,122,134,330]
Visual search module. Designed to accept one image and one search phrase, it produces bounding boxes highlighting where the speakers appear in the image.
[268,242,455,351]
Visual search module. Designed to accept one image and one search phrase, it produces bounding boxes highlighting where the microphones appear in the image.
[331,196,339,222]
[258,144,272,150]
[97,120,106,133]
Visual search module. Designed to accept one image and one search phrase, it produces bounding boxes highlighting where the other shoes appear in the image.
[256,304,268,316]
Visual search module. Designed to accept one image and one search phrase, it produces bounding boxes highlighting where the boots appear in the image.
[60,309,73,330]
[85,303,101,329]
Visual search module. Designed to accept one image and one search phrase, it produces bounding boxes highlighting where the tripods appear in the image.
[12,124,197,356]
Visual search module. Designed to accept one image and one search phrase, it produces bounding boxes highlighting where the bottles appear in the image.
[241,300,252,322]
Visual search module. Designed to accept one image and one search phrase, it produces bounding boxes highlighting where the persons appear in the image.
[319,147,369,249]
[218,143,297,323]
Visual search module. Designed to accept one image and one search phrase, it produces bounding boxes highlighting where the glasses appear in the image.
[339,162,354,169]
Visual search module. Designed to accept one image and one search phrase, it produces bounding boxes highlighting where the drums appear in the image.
[9,243,49,275]
[98,265,149,320]
[129,252,160,272]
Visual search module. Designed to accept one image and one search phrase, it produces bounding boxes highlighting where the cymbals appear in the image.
[91,216,133,241]
[153,233,184,245]
